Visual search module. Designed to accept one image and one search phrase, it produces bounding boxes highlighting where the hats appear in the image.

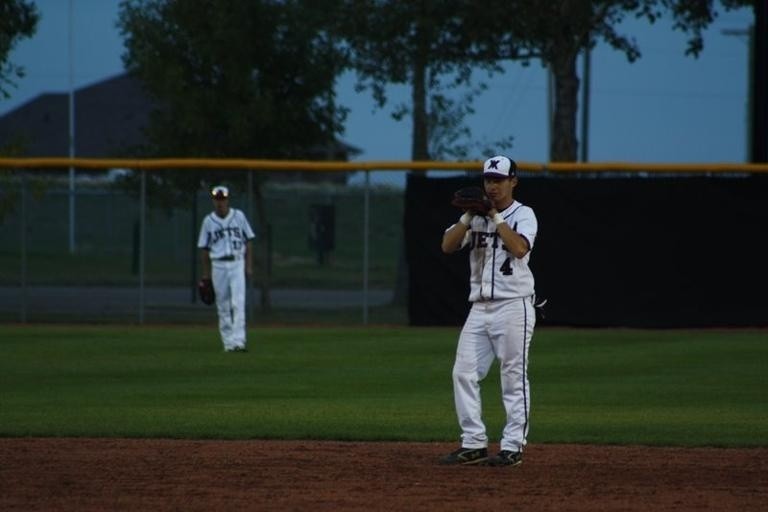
[483,156,516,179]
[210,187,230,199]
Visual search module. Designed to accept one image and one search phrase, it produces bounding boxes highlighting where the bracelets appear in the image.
[492,212,507,226]
[459,211,472,227]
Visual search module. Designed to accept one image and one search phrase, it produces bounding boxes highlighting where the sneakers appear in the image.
[437,448,488,465]
[224,346,235,351]
[234,344,246,352]
[489,450,522,467]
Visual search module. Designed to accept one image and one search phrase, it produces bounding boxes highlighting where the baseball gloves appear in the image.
[452,186,495,216]
[197,276,215,306]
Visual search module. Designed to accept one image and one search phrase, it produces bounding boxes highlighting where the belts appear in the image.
[216,256,234,261]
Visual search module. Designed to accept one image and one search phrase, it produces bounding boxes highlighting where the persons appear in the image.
[195,184,257,355]
[440,154,541,469]
